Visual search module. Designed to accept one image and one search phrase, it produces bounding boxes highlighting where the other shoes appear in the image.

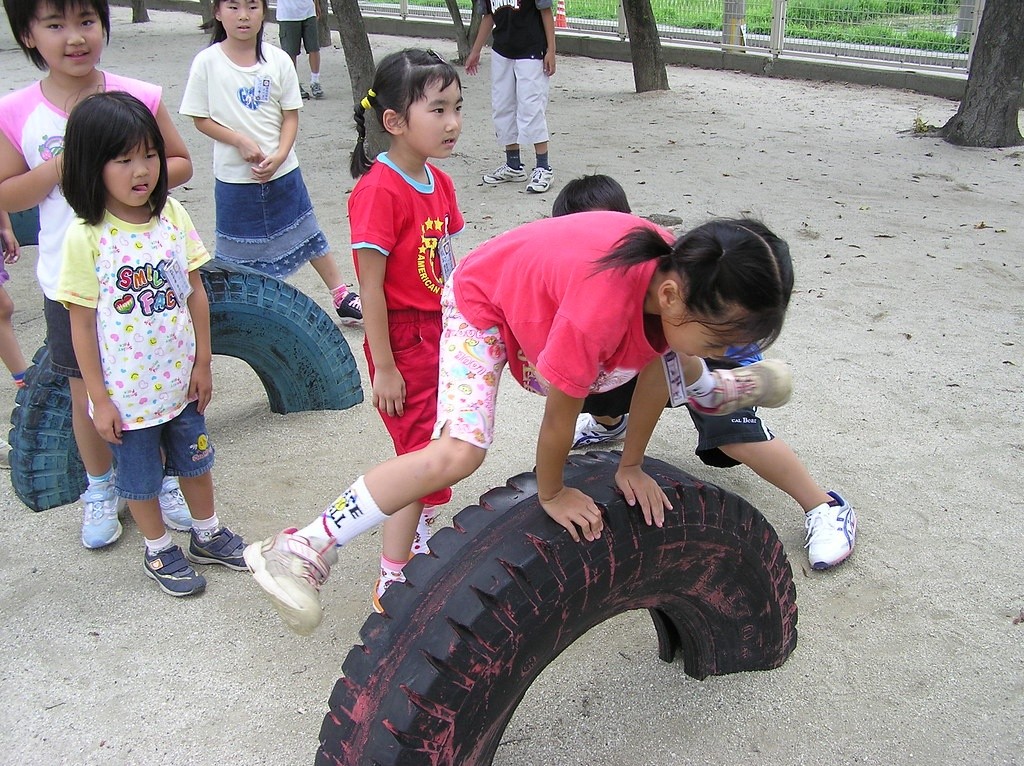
[373,579,385,614]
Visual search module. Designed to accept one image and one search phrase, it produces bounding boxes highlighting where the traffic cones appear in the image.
[555,0,567,28]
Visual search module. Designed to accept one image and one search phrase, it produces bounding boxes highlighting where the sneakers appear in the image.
[188,526,250,571]
[143,545,207,597]
[802,490,856,570]
[299,83,309,99]
[0,438,13,469]
[157,474,193,533]
[243,526,338,636]
[482,163,527,184]
[526,166,554,192]
[333,292,364,326]
[80,473,127,549]
[310,82,324,99]
[570,412,629,449]
[688,359,792,417]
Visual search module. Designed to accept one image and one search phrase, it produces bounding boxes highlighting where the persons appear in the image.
[59,91,246,596]
[2,0,216,550]
[176,1,369,327]
[1,197,31,387]
[276,0,325,99]
[243,210,795,635]
[347,48,465,615]
[551,174,858,570]
[464,0,563,194]
